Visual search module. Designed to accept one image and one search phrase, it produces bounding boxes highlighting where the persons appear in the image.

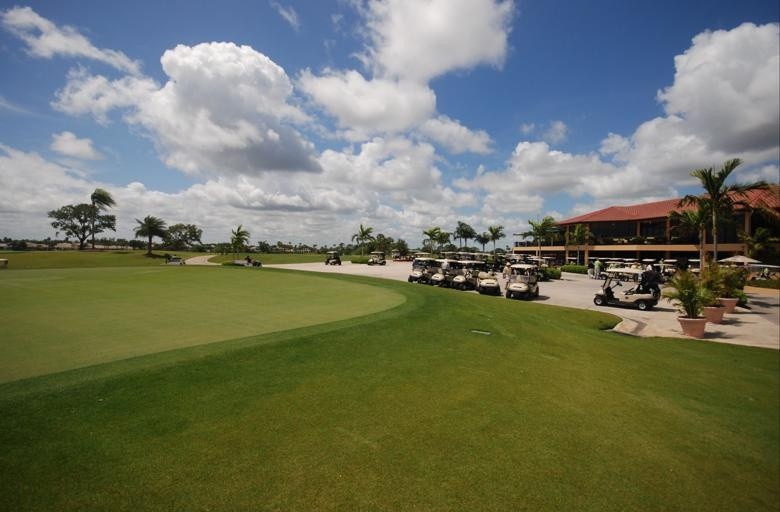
[592,257,602,280]
[502,261,511,291]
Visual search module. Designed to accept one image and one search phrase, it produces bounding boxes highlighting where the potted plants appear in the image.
[662,259,752,341]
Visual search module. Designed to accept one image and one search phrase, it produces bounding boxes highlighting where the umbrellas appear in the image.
[716,254,763,266]
